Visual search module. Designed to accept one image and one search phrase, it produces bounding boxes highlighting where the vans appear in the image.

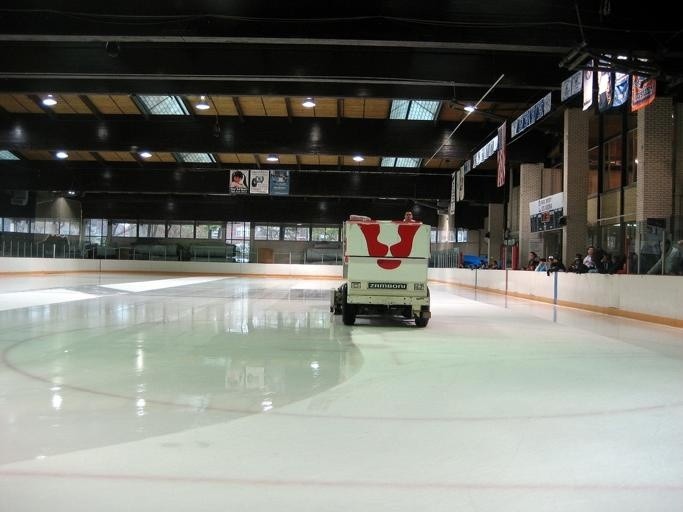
[234,244,249,259]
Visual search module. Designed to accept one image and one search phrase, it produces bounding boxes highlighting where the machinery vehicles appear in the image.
[330,211,433,328]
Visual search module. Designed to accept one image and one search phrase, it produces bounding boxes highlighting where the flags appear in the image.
[496,122,507,188]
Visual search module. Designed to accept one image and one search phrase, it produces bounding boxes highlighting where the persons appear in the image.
[647,239,683,275]
[232,171,248,190]
[403,210,420,223]
[458,245,627,276]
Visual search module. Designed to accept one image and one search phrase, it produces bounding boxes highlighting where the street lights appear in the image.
[58,196,83,250]
